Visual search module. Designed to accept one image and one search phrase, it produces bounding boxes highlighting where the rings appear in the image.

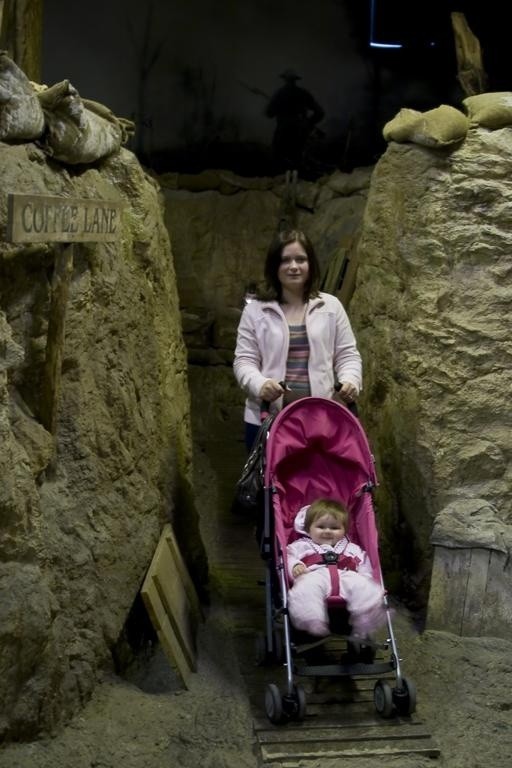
[349,394,353,400]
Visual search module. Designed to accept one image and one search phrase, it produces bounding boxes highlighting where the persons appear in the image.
[285,498,392,640]
[264,68,326,174]
[232,228,364,667]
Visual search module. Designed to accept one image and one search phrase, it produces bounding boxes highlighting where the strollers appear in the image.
[259,381,416,724]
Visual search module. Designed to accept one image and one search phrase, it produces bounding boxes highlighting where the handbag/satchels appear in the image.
[232,443,267,514]
